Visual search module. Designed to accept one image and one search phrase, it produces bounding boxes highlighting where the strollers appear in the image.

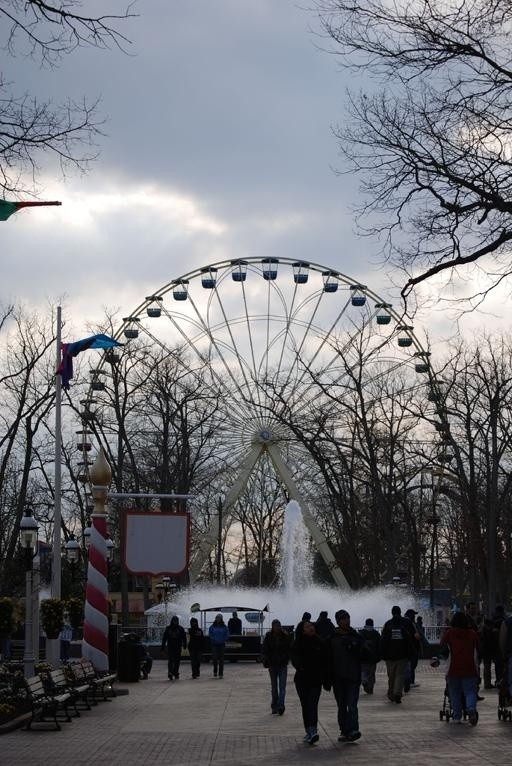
[438,662,482,724]
[495,660,512,721]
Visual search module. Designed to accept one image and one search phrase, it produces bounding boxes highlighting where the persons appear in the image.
[130,632,153,680]
[188,618,205,680]
[162,616,187,680]
[261,602,507,746]
[228,611,242,635]
[209,614,228,679]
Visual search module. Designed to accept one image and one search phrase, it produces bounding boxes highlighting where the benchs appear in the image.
[27,661,117,730]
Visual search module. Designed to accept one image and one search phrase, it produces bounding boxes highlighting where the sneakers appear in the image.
[468,706,477,725]
[303,727,319,744]
[337,729,361,741]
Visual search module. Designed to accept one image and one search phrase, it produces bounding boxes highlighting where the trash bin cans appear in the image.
[116,644,143,682]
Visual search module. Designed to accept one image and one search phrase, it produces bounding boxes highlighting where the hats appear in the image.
[404,610,419,617]
[335,609,350,624]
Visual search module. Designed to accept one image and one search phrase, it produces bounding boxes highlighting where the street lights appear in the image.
[69,521,116,612]
[154,574,180,624]
[17,508,40,675]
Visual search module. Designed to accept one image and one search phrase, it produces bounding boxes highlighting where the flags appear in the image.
[57,335,126,387]
